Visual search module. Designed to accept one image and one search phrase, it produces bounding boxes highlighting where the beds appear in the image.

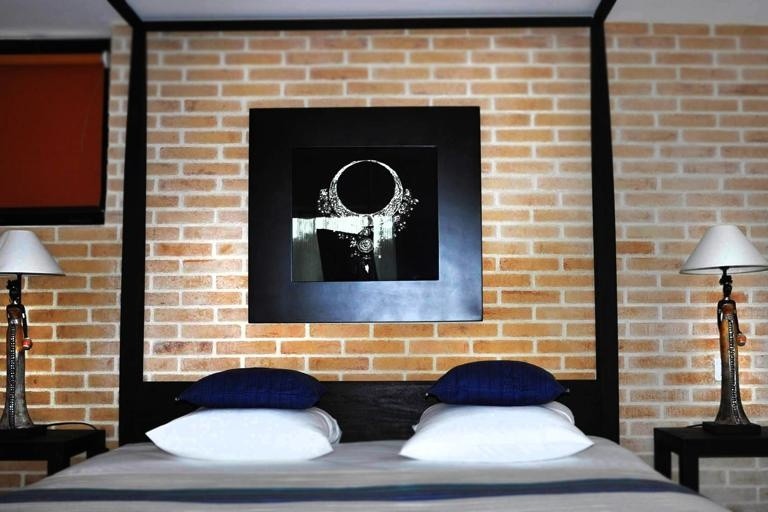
[0,381,730,512]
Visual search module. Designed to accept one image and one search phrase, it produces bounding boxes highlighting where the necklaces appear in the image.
[316,160,421,276]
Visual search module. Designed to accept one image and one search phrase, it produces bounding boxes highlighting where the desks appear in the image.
[0,428,106,475]
[653,425,768,494]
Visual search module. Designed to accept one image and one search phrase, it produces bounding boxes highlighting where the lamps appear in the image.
[0,230,67,434]
[680,224,768,433]
